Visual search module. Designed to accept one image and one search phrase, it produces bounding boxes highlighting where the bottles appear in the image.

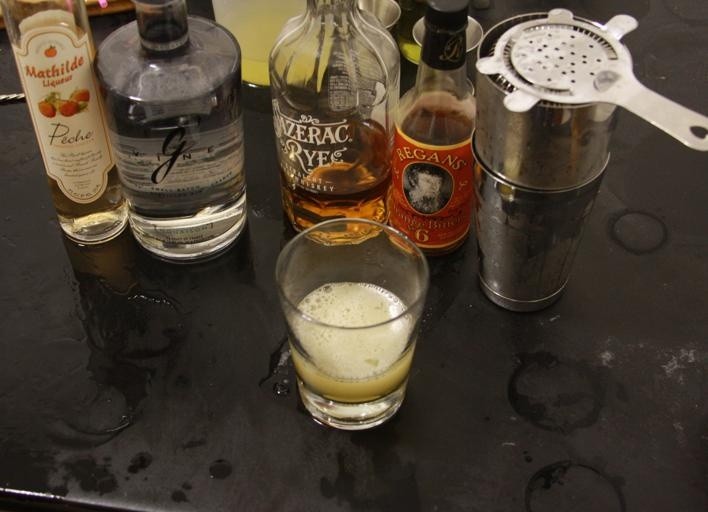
[478,10,708,190]
[260,0,401,244]
[2,2,129,250]
[390,2,474,253]
[95,0,247,266]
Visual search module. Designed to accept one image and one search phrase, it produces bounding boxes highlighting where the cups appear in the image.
[412,9,483,59]
[473,146,612,315]
[274,216,430,432]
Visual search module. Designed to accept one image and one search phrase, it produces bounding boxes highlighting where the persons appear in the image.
[405,163,446,213]
